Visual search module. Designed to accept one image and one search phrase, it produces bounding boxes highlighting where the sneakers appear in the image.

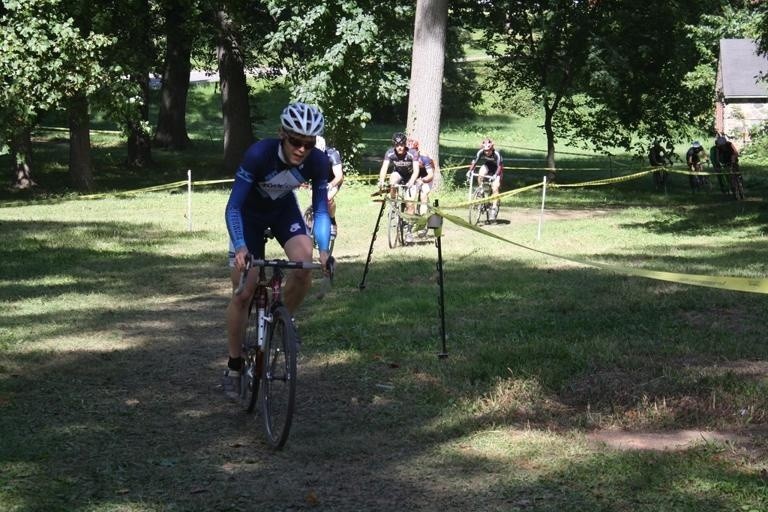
[223,368,240,399]
[277,316,299,353]
[404,232,413,242]
[330,224,336,241]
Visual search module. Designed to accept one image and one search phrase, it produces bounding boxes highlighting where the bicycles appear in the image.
[650,162,673,195]
[374,184,433,248]
[225,253,335,451]
[715,164,730,195]
[685,159,713,195]
[303,205,334,255]
[467,172,502,226]
[718,162,745,201]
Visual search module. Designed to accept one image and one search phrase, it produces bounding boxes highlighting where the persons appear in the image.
[376,132,419,244]
[649,137,739,195]
[311,135,344,239]
[405,138,435,236]
[224,104,337,401]
[466,138,504,221]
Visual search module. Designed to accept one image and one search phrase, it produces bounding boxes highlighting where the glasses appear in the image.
[286,133,316,149]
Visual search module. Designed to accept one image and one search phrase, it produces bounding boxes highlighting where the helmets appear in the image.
[314,135,325,152]
[480,139,494,150]
[391,134,406,144]
[279,103,325,136]
[405,138,418,149]
